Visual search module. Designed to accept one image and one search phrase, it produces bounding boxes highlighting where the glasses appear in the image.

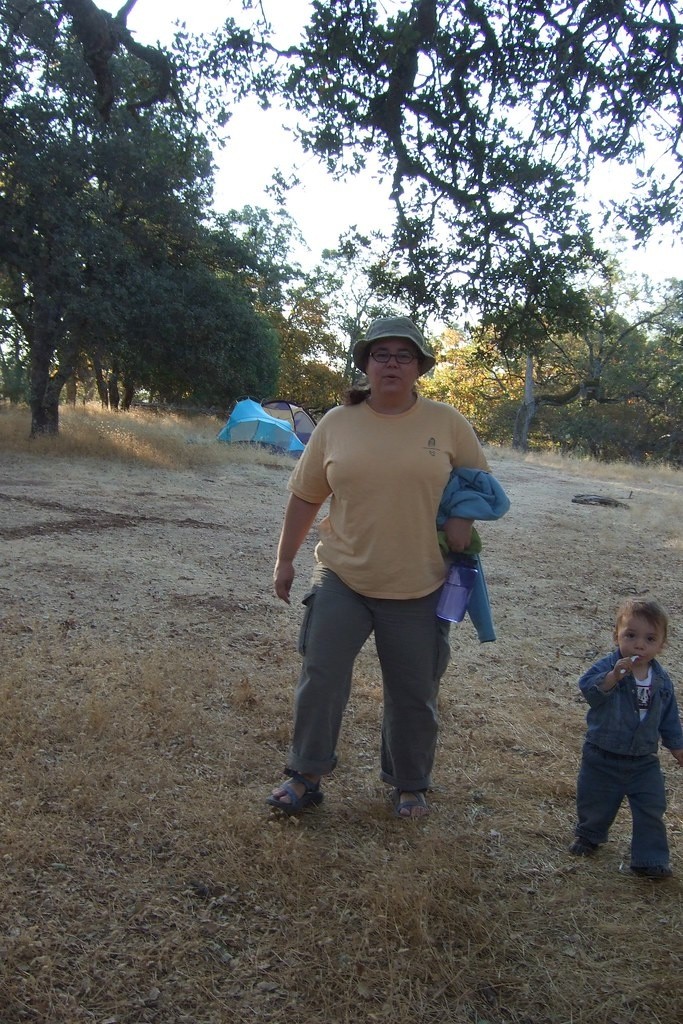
[366,350,420,364]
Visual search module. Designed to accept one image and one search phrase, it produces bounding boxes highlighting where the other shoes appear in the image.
[568,835,598,857]
[630,865,673,879]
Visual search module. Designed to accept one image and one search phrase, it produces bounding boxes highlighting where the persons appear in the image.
[568,596,683,877]
[266,317,489,820]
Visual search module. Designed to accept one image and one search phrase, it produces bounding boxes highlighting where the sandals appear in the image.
[395,785,429,817]
[264,773,323,812]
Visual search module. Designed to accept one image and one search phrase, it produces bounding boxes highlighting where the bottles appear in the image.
[437,555,479,624]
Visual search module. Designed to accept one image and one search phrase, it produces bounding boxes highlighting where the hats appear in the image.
[352,317,436,380]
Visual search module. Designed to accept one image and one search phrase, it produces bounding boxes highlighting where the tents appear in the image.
[216,398,318,457]
